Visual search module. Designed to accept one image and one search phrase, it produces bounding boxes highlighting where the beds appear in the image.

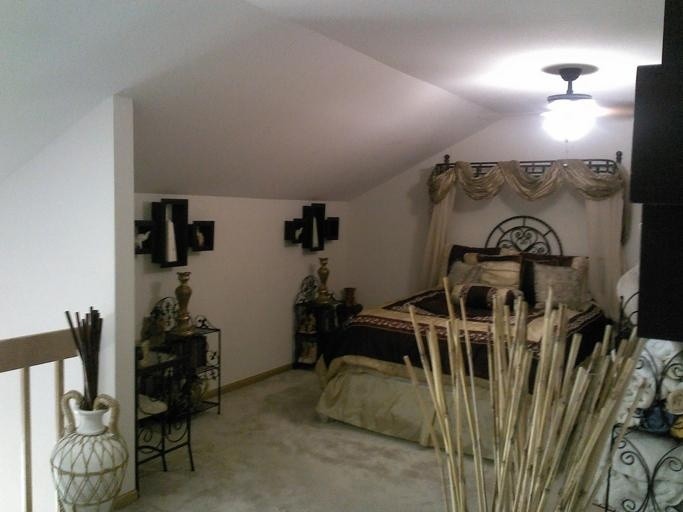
[314,151,632,461]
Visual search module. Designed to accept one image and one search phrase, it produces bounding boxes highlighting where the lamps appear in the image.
[545,68,597,142]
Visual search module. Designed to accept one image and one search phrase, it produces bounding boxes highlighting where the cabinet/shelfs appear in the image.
[294,275,346,373]
[142,297,222,415]
[136,349,195,498]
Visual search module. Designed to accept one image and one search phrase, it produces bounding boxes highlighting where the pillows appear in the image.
[436,241,591,311]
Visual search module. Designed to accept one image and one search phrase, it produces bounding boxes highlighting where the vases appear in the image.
[174,271,193,328]
[50,390,130,512]
[315,258,330,305]
